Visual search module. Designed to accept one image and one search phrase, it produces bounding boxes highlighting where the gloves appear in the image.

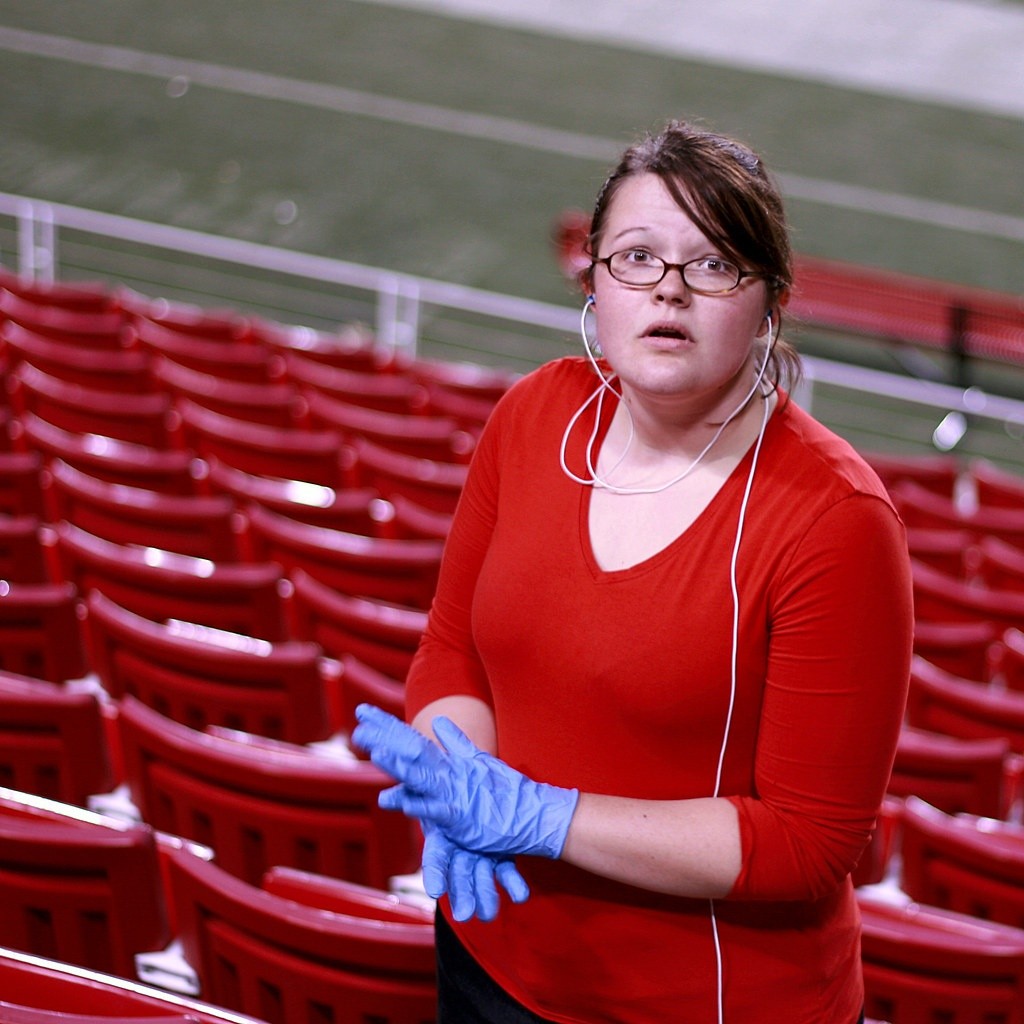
[351,702,579,859]
[377,782,529,922]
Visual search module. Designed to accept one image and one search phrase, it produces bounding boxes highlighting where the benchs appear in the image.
[558,211,1024,390]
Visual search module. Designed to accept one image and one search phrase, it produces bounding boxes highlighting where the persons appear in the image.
[353,116,914,1024]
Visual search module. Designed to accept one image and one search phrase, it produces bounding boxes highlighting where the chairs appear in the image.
[0,270,1024,1024]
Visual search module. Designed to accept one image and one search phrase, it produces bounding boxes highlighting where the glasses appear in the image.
[592,249,769,294]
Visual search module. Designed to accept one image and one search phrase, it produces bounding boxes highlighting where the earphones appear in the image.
[766,308,773,320]
[589,292,596,306]
